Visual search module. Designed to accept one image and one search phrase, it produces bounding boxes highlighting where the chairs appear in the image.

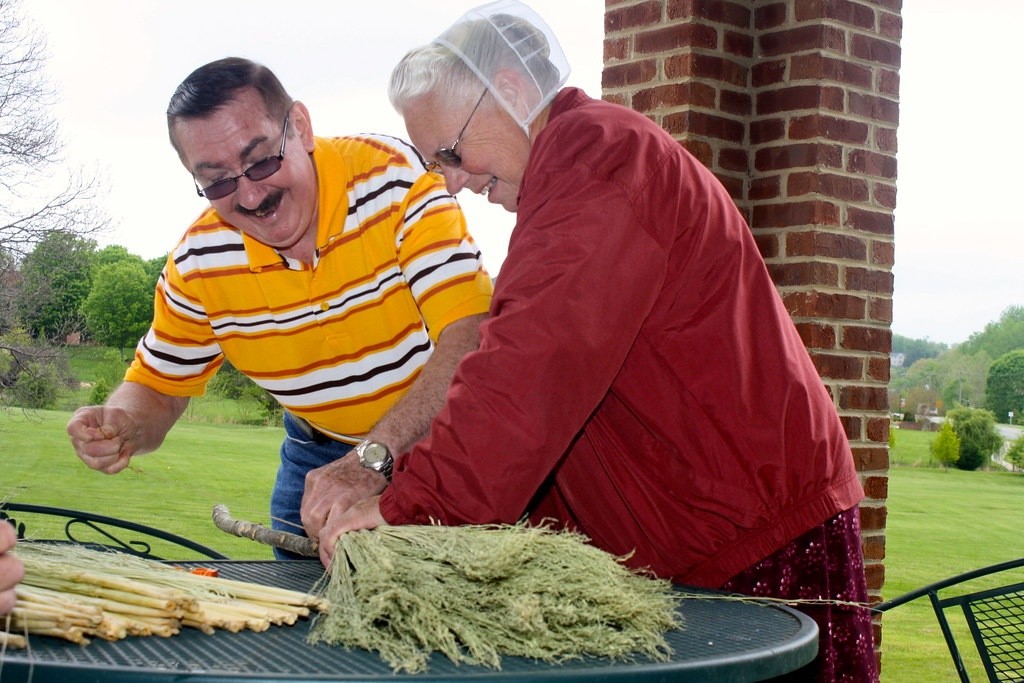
[0,503,230,561]
[869,557,1024,683]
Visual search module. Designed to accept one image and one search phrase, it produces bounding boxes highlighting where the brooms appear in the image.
[213,504,689,680]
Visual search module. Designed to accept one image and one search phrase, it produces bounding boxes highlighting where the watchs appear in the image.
[346,435,397,479]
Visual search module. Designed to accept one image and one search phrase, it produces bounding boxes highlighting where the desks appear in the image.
[0,560,819,683]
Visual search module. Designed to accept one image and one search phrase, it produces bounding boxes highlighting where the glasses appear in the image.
[426,88,488,174]
[192,112,288,200]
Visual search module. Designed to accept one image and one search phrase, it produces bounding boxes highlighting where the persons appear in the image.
[67,55,498,558]
[0,517,27,615]
[317,17,885,683]
